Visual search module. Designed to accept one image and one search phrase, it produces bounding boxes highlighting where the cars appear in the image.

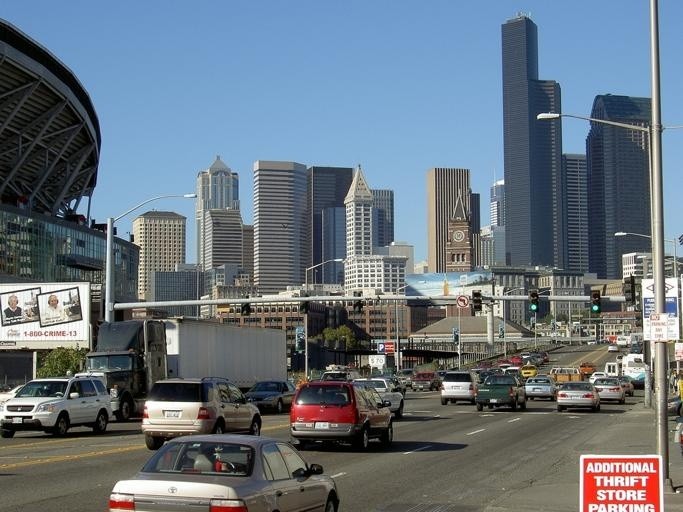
[587,333,644,353]
[104,430,343,512]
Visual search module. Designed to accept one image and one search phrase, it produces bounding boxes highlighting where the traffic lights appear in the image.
[589,289,601,313]
[528,288,539,313]
[471,289,483,312]
[632,301,643,327]
[622,273,635,303]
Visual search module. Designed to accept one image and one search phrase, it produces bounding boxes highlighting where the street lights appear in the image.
[564,291,573,346]
[304,258,344,378]
[533,290,555,350]
[503,286,525,358]
[611,231,681,376]
[530,108,652,243]
[396,279,427,372]
[103,193,199,321]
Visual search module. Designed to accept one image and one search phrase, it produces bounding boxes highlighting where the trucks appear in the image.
[84,317,290,424]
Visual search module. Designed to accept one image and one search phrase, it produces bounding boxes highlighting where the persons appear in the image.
[43,295,64,323]
[3,294,25,323]
